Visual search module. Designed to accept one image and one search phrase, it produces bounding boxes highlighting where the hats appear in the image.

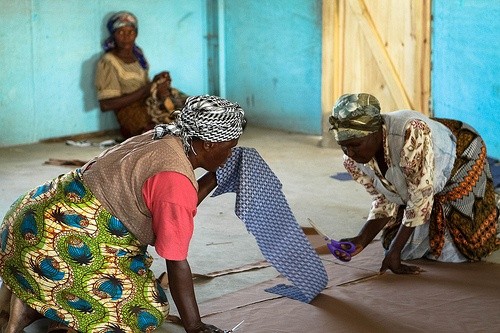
[153,95,247,153]
[330,94,384,142]
[106,11,141,35]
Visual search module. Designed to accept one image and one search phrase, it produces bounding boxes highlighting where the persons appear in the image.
[328,93,500,275]
[94,11,190,138]
[0,95,245,333]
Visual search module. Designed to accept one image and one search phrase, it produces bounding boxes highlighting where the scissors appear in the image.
[214,319,245,332]
[308,218,356,263]
